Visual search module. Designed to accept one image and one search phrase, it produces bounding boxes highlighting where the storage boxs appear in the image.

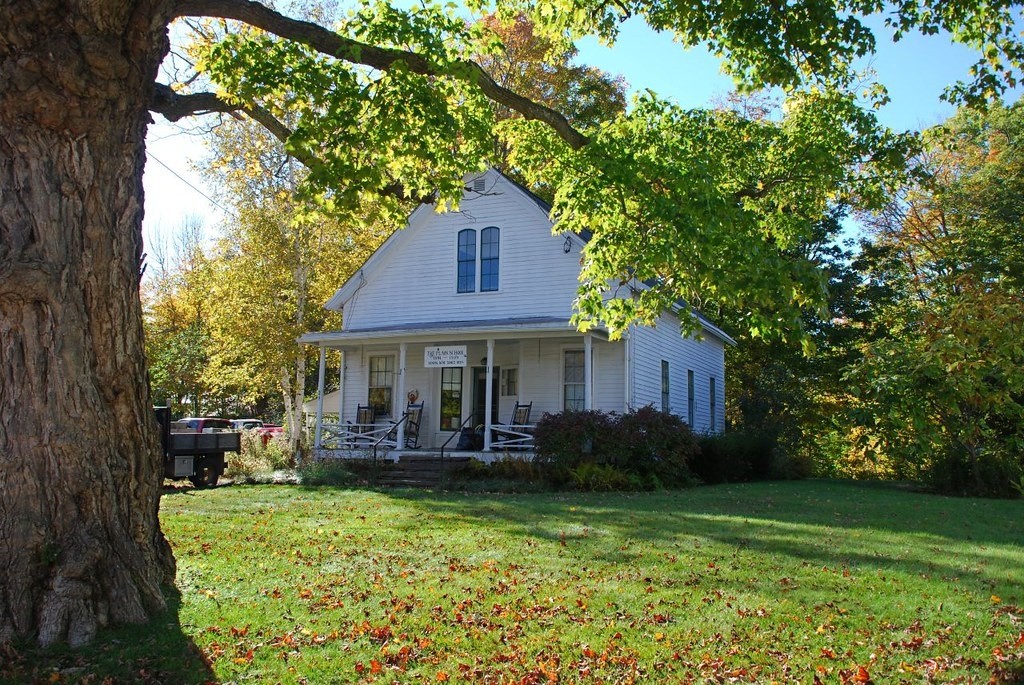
[166,456,194,477]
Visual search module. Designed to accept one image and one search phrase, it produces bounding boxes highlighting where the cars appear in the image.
[151,405,286,492]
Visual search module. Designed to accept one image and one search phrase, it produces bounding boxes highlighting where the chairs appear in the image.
[347,403,377,449]
[387,400,424,449]
[492,401,532,451]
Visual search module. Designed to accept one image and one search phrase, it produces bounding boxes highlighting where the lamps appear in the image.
[480,357,487,365]
